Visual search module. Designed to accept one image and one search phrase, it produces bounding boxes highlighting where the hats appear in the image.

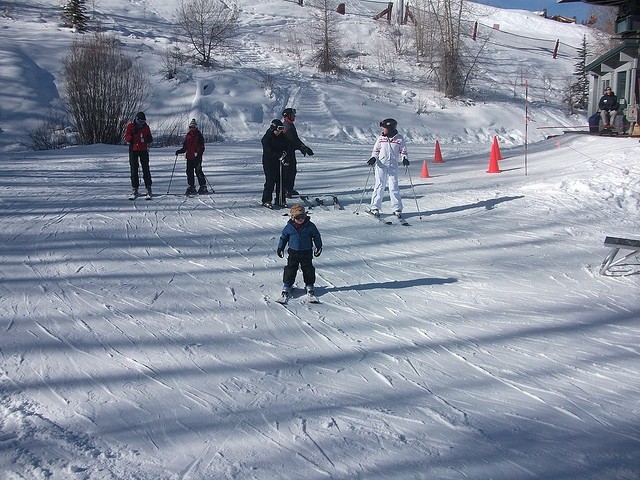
[190,119,197,126]
[290,204,305,220]
[270,119,284,131]
[136,112,146,120]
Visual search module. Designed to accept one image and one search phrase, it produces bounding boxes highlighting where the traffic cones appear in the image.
[432,140,445,163]
[486,145,502,173]
[419,160,431,178]
[493,136,504,160]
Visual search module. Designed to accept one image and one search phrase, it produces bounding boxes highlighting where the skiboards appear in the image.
[259,202,309,216]
[365,210,409,225]
[314,196,345,212]
[129,190,153,200]
[275,283,320,303]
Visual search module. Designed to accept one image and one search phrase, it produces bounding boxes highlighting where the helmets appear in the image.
[282,108,295,120]
[380,119,398,137]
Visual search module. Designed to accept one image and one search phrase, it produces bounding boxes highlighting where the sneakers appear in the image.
[283,284,293,292]
[394,211,401,215]
[305,283,314,292]
[371,209,379,214]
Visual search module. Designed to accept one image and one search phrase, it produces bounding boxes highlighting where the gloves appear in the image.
[306,147,314,156]
[279,150,287,161]
[403,159,409,166]
[314,247,322,257]
[281,159,290,166]
[277,249,284,258]
[366,157,376,165]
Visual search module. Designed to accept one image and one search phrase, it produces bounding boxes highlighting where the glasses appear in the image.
[294,215,306,220]
[137,119,145,125]
[272,123,284,131]
[189,126,195,128]
[291,109,296,113]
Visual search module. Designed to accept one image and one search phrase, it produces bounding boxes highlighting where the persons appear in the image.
[260,118,288,207]
[175,118,209,195]
[282,107,315,198]
[277,204,324,298]
[124,110,154,195]
[599,86,618,131]
[366,119,409,217]
